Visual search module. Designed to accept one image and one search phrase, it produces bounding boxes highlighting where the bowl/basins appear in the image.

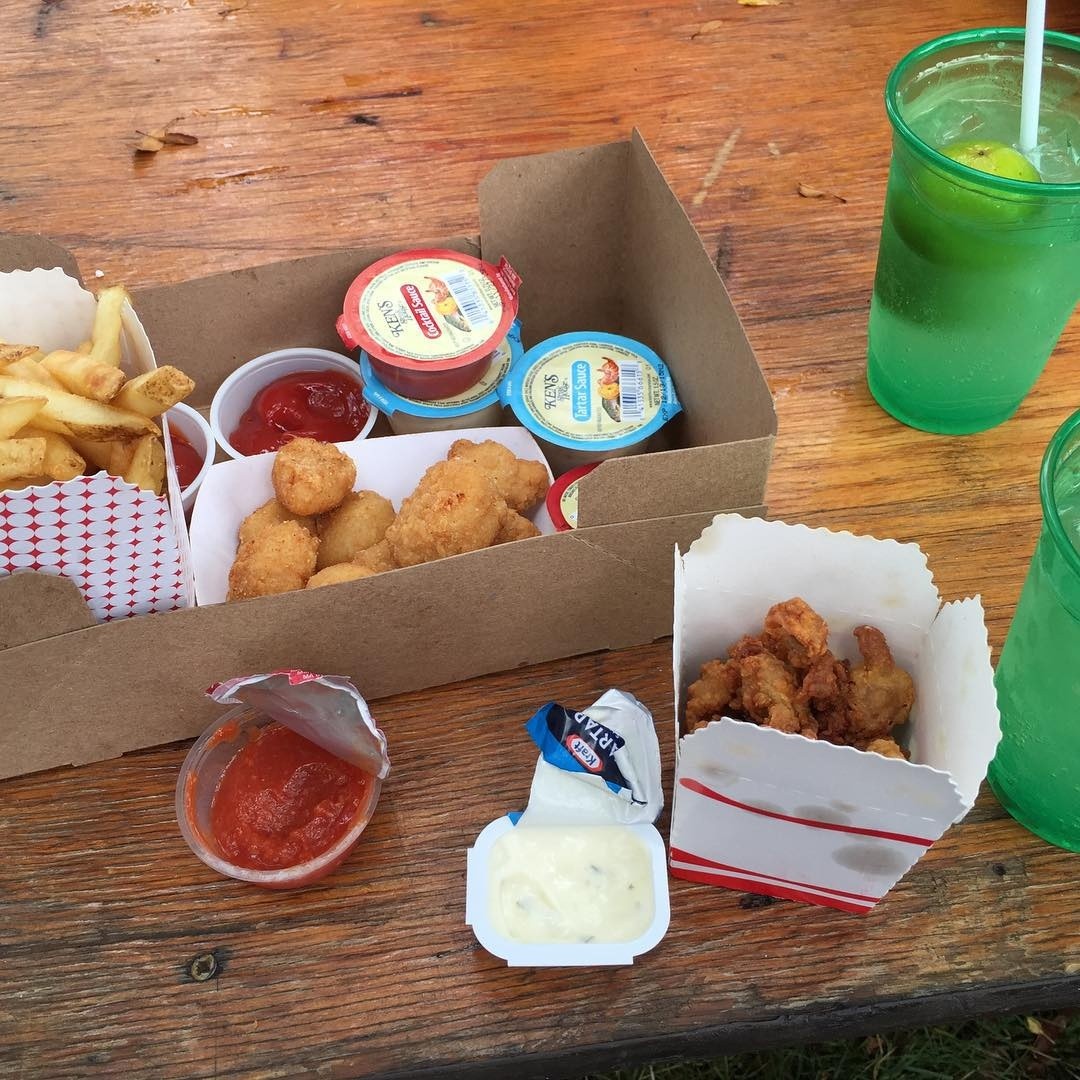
[340,247,683,532]
[174,700,381,888]
[466,816,669,966]
[166,402,215,523]
[209,346,379,458]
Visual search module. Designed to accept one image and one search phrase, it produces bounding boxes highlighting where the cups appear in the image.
[863,26,1080,437]
[988,410,1079,853]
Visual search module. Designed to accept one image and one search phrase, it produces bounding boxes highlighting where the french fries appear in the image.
[1,284,197,495]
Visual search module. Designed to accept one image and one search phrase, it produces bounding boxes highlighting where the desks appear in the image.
[0,0,1080,1080]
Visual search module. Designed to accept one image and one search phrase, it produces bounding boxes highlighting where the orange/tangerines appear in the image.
[876,141,1042,325]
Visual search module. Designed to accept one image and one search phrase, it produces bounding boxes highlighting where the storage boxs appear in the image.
[668,513,1003,917]
[0,125,777,778]
